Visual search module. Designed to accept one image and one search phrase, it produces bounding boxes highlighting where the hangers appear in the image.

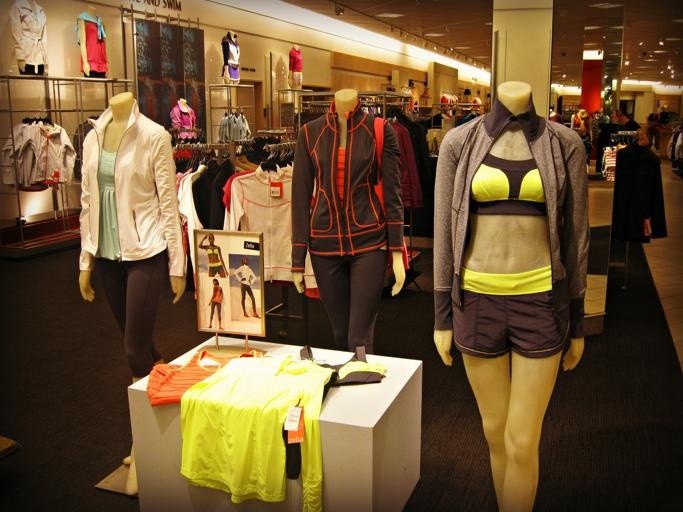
[19,107,55,128]
[165,134,297,181]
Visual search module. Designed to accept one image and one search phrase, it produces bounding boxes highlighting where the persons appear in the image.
[599,111,610,124]
[11,1,50,75]
[199,233,229,278]
[234,257,259,318]
[77,6,111,77]
[618,112,641,146]
[221,30,242,83]
[169,98,198,144]
[289,88,407,355]
[78,93,186,496]
[288,44,304,88]
[550,108,563,124]
[570,109,591,172]
[433,82,592,512]
[208,279,224,330]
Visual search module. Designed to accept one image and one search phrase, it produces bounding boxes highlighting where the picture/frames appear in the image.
[192,230,266,339]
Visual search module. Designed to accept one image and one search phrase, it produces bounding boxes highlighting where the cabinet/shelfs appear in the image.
[278,89,313,138]
[208,84,255,156]
[0,74,134,258]
